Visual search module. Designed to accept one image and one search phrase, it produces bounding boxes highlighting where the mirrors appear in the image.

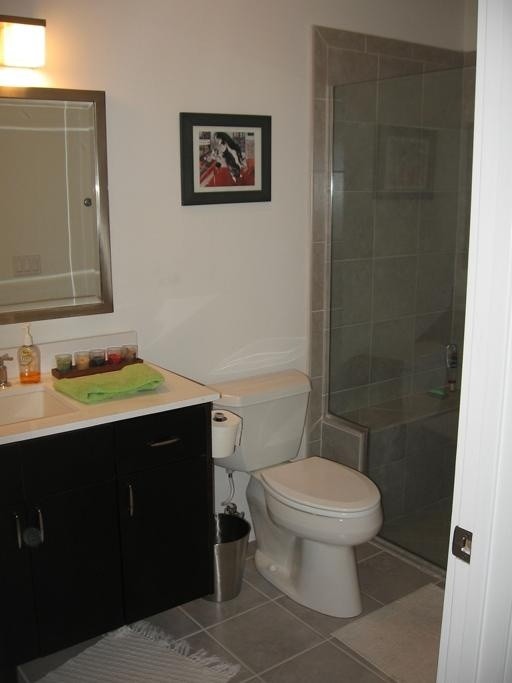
[0,84,113,323]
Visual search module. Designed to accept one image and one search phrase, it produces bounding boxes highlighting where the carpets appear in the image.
[328,582,443,683]
[32,619,241,682]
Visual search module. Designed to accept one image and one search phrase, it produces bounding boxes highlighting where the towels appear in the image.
[53,361,165,405]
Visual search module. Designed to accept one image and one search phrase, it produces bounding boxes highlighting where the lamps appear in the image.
[0,15,47,69]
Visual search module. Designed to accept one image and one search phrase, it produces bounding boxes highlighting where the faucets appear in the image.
[0,352,14,388]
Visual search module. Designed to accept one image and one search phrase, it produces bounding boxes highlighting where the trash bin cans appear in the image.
[202,513,252,602]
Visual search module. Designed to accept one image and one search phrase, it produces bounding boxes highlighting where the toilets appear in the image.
[204,367,386,621]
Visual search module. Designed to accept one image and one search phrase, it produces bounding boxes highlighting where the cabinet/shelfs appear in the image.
[0,422,125,672]
[114,402,213,628]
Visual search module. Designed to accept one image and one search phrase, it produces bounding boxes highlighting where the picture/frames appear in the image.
[179,112,272,206]
[370,124,439,202]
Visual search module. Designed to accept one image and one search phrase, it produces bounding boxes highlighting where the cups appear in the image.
[55,353,72,370]
[73,344,138,370]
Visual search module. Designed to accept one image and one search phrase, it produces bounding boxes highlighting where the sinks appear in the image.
[0,383,81,426]
